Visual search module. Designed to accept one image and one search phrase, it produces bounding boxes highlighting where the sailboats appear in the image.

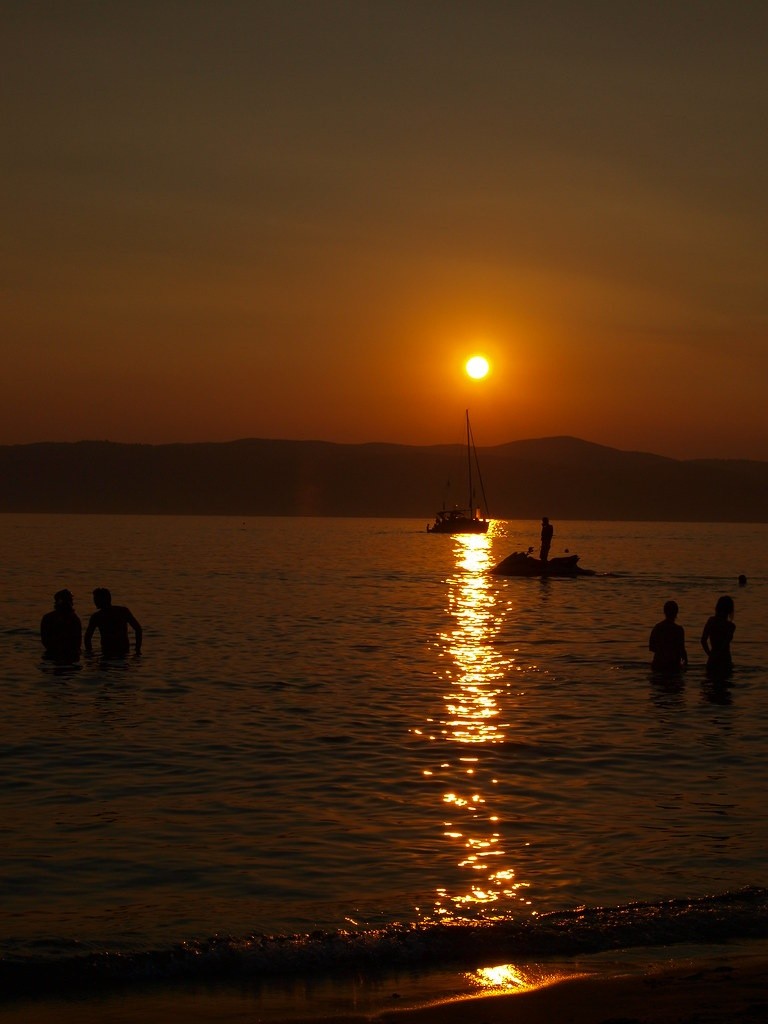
[424,407,490,534]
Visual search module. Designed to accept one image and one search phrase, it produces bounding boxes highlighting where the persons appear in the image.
[539,517,553,562]
[429,512,456,528]
[40,589,82,664]
[738,574,747,587]
[700,596,736,680]
[84,587,142,655]
[649,600,688,673]
